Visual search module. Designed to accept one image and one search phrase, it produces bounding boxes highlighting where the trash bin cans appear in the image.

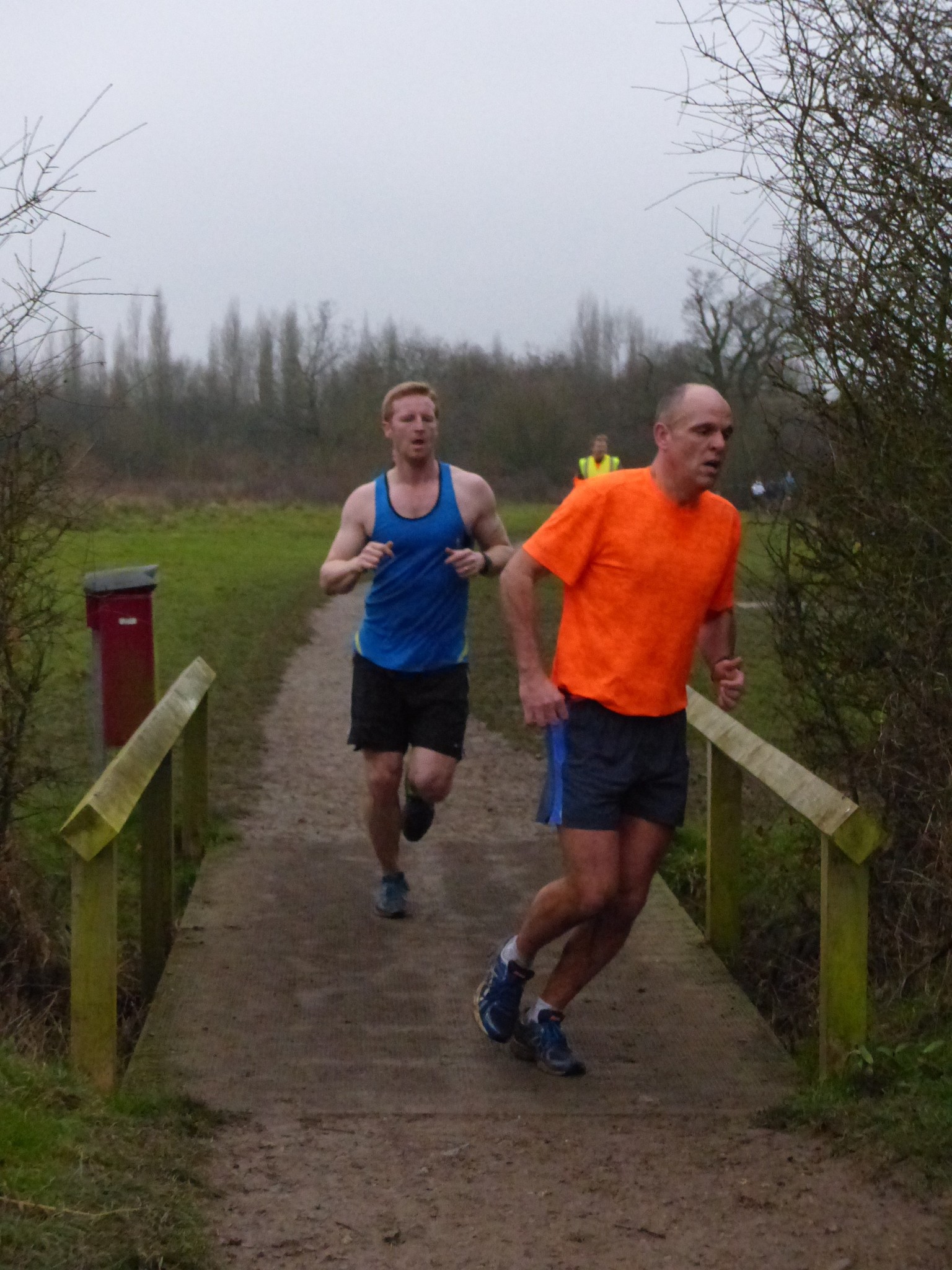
[82,564,162,751]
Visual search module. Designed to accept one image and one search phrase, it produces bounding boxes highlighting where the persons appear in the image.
[471,381,745,1081]
[317,380,515,921]
[572,433,625,488]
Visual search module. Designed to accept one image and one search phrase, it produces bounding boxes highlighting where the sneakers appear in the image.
[402,777,434,842]
[511,1006,585,1076]
[375,872,409,919]
[474,933,535,1044]
